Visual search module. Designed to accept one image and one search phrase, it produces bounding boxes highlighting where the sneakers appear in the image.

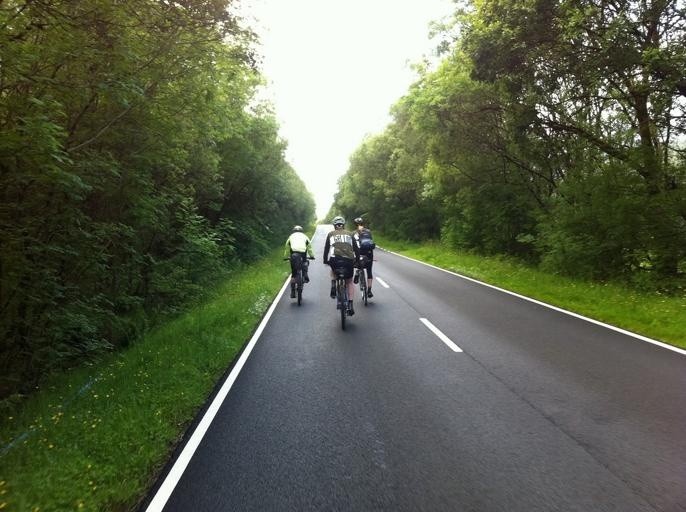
[366,291,374,298]
[347,307,355,317]
[290,290,295,298]
[330,286,337,299]
[353,274,359,284]
[303,274,310,283]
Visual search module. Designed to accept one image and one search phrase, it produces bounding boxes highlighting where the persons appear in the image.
[323,216,373,316]
[284,225,315,298]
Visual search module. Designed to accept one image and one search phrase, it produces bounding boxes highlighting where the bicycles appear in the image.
[283,257,315,305]
[323,257,373,330]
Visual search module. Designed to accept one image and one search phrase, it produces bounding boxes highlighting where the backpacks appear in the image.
[356,228,376,250]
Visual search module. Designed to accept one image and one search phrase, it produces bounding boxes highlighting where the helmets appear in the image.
[293,225,304,232]
[332,216,345,226]
[354,217,364,225]
